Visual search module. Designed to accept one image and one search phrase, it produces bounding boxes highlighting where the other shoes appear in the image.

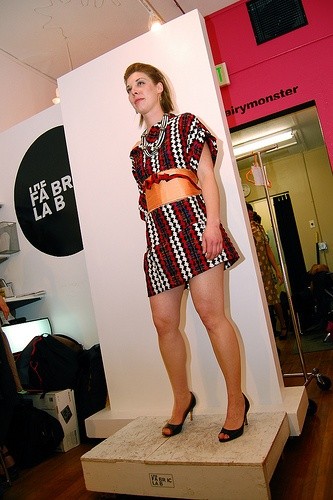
[2,448,17,479]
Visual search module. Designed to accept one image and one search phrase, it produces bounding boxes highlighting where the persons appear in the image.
[124,63,251,442]
[246,203,284,358]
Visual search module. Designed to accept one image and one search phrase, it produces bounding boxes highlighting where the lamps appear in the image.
[233,127,297,161]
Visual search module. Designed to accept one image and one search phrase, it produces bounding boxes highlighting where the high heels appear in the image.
[219,393,249,443]
[279,324,289,340]
[161,392,196,437]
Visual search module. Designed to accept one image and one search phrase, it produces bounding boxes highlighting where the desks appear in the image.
[3,296,41,319]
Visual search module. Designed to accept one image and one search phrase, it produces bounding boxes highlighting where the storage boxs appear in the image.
[18,389,80,453]
